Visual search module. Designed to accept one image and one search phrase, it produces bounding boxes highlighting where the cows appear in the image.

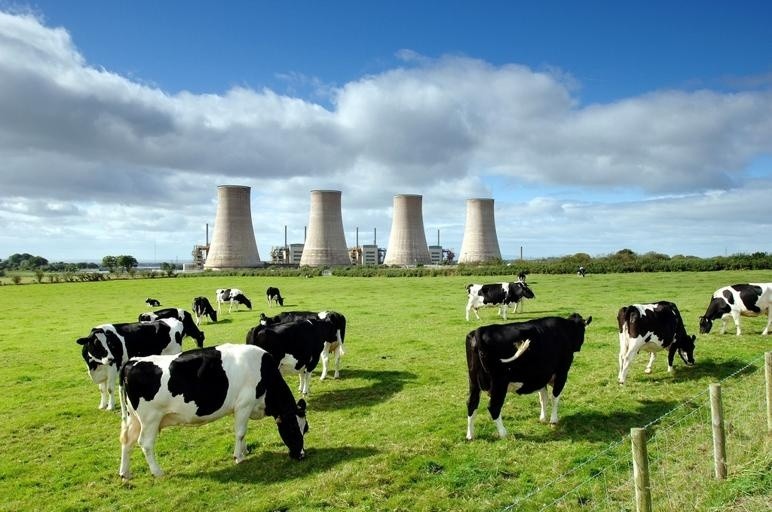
[191,296,217,326]
[616,301,697,385]
[266,286,284,307]
[118,343,309,480]
[465,280,535,322]
[215,288,253,315]
[464,312,593,442]
[698,281,772,337]
[517,271,527,282]
[577,266,586,277]
[76,307,205,412]
[246,310,346,395]
[145,296,161,307]
